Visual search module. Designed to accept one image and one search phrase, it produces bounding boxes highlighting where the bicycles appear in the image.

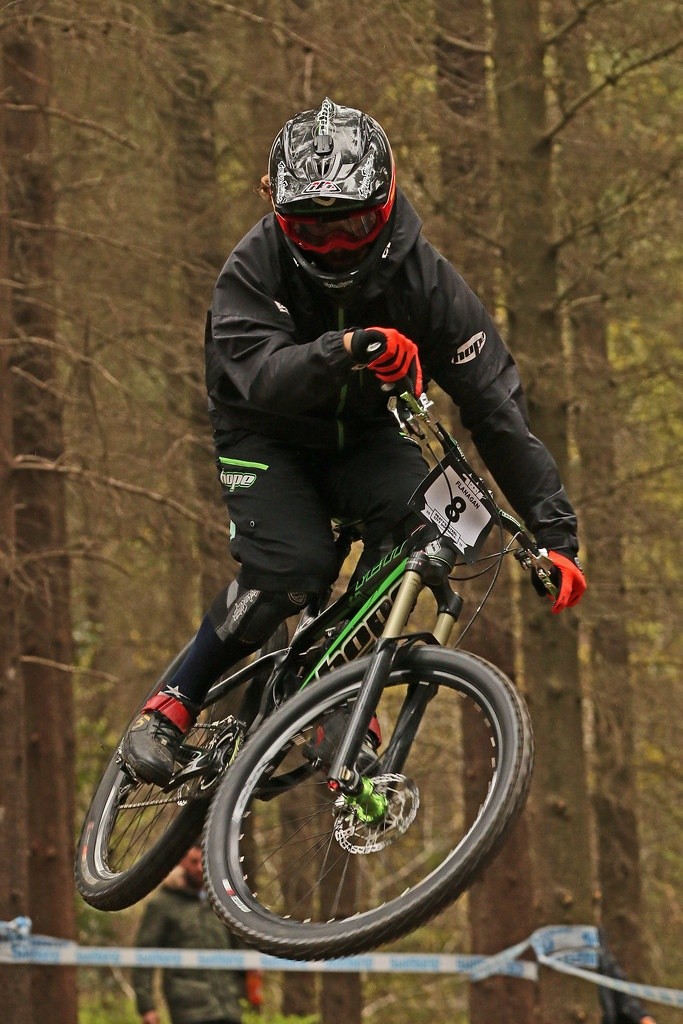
[74,342,565,961]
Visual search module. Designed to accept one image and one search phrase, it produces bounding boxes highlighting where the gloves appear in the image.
[350,325,423,400]
[531,550,588,615]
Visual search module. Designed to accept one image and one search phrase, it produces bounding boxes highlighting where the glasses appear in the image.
[275,202,393,254]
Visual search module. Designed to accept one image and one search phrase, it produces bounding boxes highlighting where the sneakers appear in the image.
[301,703,383,777]
[122,694,195,788]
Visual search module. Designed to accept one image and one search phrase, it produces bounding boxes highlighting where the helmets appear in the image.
[267,96,397,293]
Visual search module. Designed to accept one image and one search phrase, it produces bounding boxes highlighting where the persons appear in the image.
[132,835,262,1024]
[121,97,588,787]
